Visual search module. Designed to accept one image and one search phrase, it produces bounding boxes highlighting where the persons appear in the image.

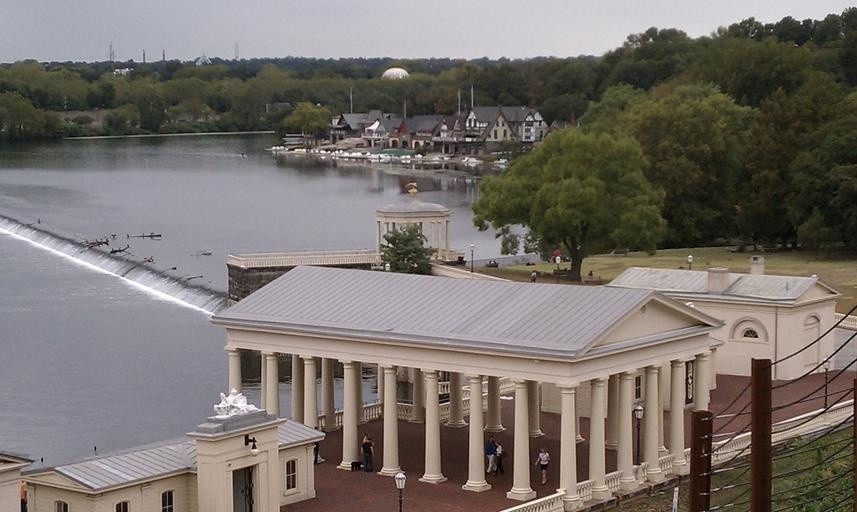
[492,441,504,475]
[526,255,571,266]
[485,436,497,474]
[220,388,258,417]
[531,271,537,283]
[360,438,376,472]
[314,427,319,464]
[534,447,551,485]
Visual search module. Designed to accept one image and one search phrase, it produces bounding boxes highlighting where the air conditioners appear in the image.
[351,461,364,471]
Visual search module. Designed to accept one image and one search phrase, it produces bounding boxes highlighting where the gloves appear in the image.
[264,145,510,172]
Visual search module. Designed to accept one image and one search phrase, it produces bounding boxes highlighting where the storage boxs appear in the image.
[584,275,604,284]
[487,264,499,267]
[553,270,570,277]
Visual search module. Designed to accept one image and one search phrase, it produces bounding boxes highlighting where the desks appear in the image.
[394,472,406,512]
[469,243,475,272]
[555,254,561,284]
[822,357,830,409]
[687,253,693,270]
[635,404,644,466]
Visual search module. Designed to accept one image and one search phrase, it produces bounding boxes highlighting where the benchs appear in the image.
[398,120,407,140]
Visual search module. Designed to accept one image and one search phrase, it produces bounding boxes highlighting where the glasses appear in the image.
[244,433,259,456]
